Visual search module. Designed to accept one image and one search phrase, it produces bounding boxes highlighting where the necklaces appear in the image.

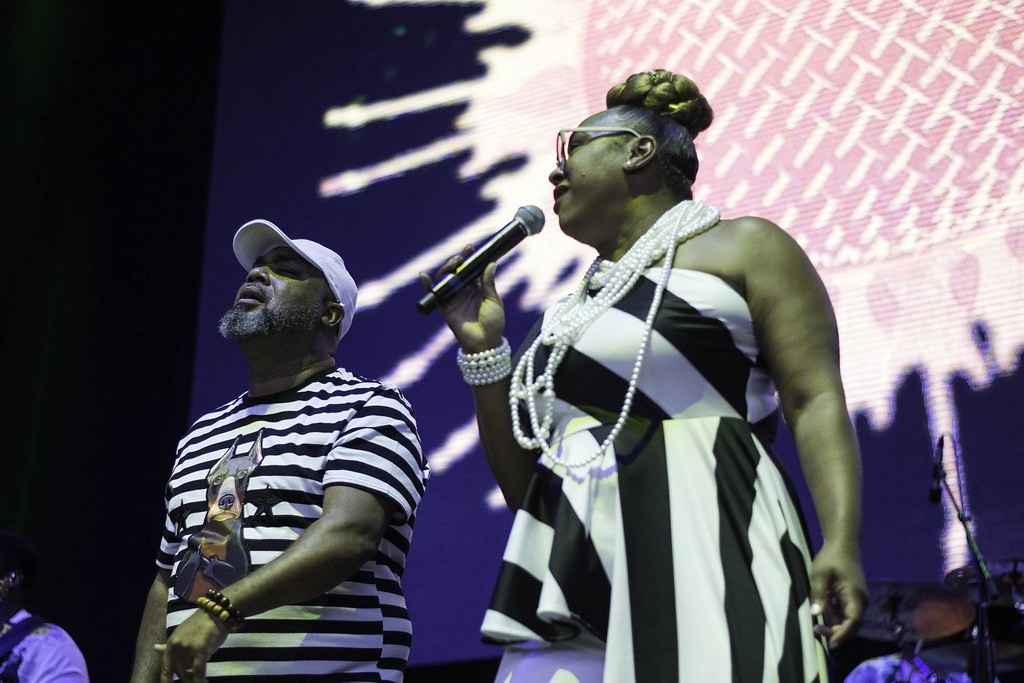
[510,199,720,468]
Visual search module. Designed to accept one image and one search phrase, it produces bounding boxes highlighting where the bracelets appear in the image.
[457,336,511,385]
[196,588,245,633]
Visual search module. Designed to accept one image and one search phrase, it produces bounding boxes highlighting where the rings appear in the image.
[186,668,194,673]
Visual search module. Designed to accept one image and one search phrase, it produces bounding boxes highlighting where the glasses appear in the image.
[556,127,642,170]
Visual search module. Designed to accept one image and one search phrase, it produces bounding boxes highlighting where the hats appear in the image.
[233,218,357,342]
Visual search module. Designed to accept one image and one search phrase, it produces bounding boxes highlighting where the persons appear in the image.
[0,531,89,683]
[418,69,868,683]
[132,220,430,683]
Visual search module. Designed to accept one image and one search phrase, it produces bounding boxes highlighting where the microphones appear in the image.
[929,435,945,502]
[417,206,545,316]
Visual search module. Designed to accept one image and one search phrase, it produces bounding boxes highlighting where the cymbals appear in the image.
[823,580,976,642]
